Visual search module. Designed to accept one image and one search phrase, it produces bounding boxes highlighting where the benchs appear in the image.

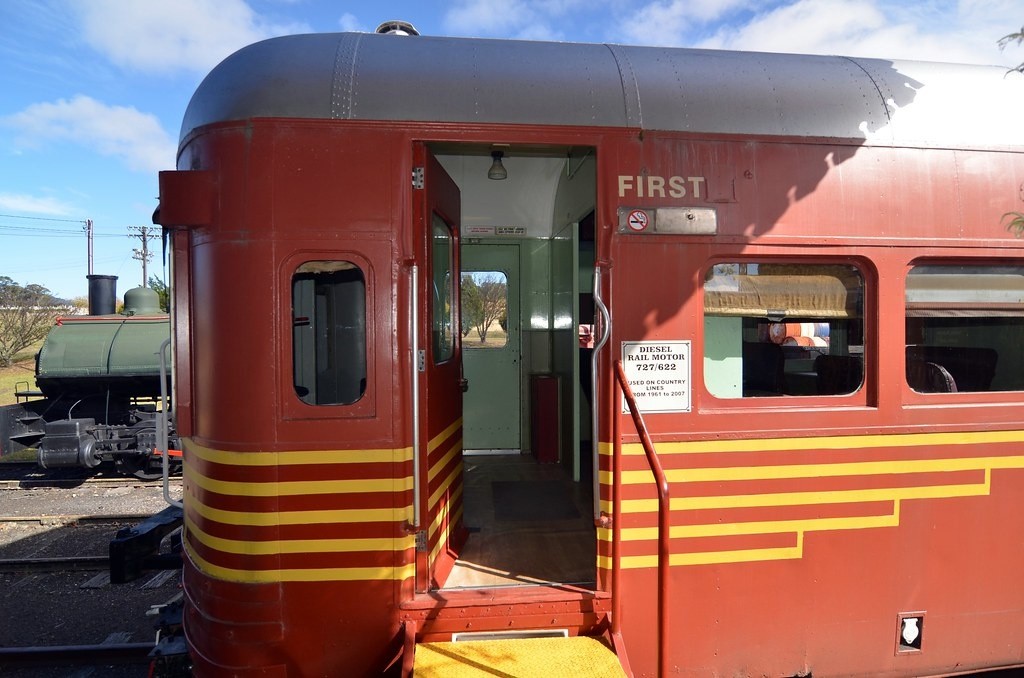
[742,341,999,397]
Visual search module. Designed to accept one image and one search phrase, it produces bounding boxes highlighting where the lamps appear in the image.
[488,150,507,180]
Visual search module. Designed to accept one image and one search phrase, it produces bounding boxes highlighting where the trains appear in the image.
[93,20,1024,678]
[4,273,182,480]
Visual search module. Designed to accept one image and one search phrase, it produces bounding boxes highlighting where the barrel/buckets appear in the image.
[783,336,829,348]
[769,323,830,343]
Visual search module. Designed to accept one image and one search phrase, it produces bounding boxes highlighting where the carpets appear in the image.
[491,479,586,521]
